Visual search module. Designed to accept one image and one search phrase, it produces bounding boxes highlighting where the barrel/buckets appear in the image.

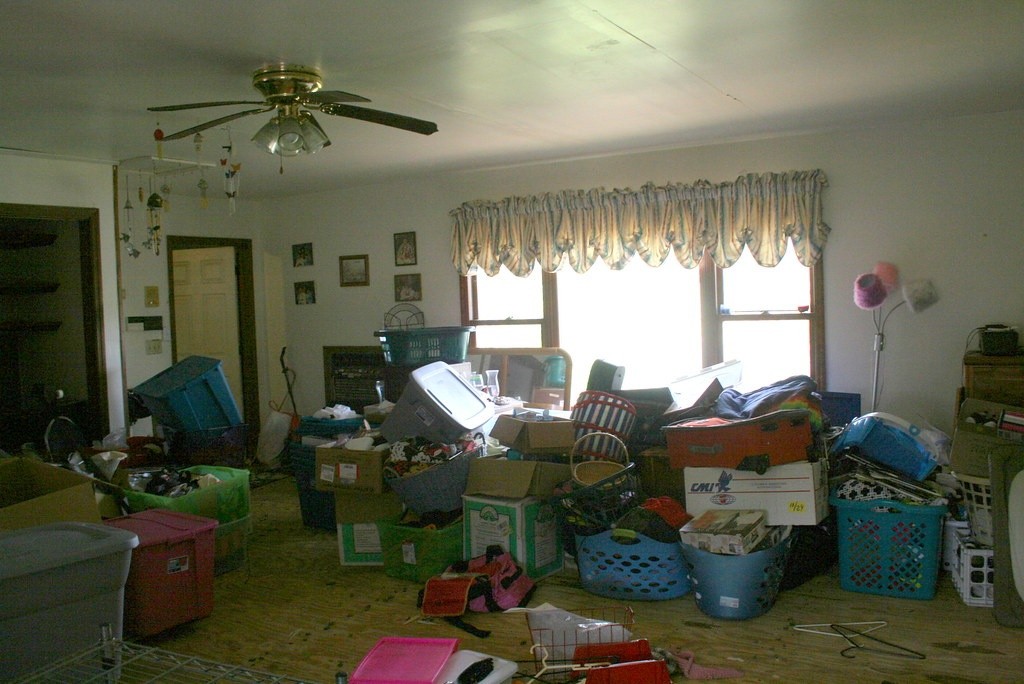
[542,356,567,388]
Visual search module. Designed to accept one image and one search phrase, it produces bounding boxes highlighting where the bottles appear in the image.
[485,369,500,398]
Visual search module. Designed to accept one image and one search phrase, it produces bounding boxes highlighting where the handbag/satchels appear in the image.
[418,546,539,636]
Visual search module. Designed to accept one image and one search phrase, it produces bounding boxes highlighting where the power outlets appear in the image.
[963,351,1023,408]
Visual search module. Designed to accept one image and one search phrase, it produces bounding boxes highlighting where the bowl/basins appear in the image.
[346,437,373,451]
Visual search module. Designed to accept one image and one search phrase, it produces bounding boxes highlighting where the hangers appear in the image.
[511,644,619,684]
[792,621,926,660]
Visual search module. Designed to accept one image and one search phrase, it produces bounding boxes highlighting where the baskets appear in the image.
[373,327,476,365]
[570,433,629,492]
[574,527,690,600]
[950,529,994,608]
[828,488,948,599]
[676,533,793,620]
[950,471,993,547]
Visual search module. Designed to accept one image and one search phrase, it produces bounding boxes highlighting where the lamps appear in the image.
[145,63,440,142]
[251,104,329,175]
[854,262,938,412]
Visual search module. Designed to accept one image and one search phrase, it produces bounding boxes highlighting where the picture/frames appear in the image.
[293,281,316,304]
[393,231,417,266]
[340,254,370,287]
[393,273,422,302]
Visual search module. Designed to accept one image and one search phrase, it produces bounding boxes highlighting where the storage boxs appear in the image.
[284,326,1024,607]
[0,355,253,683]
[530,355,567,409]
[433,650,518,684]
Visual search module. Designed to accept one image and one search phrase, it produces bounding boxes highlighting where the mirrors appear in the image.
[465,348,572,413]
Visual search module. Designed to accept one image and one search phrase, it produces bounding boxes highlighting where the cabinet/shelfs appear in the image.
[0,231,63,332]
